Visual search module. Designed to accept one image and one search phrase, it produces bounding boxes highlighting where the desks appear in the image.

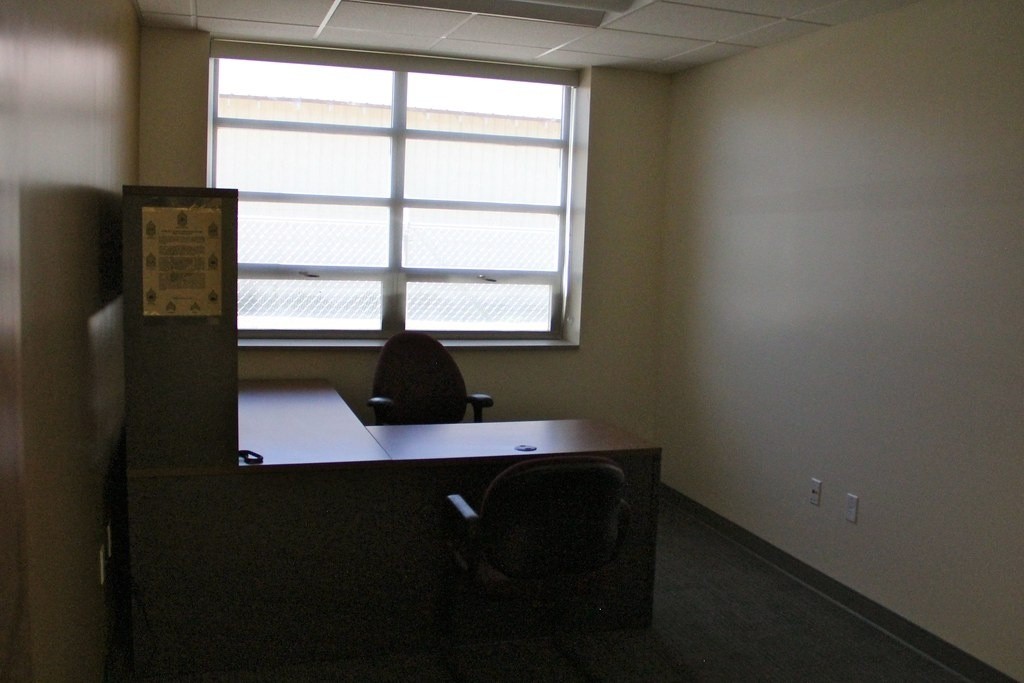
[366,418,662,657]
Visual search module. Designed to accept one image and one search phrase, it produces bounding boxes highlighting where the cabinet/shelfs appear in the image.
[122,184,391,683]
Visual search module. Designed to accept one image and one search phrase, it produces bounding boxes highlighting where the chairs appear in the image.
[426,455,626,682]
[366,331,494,426]
